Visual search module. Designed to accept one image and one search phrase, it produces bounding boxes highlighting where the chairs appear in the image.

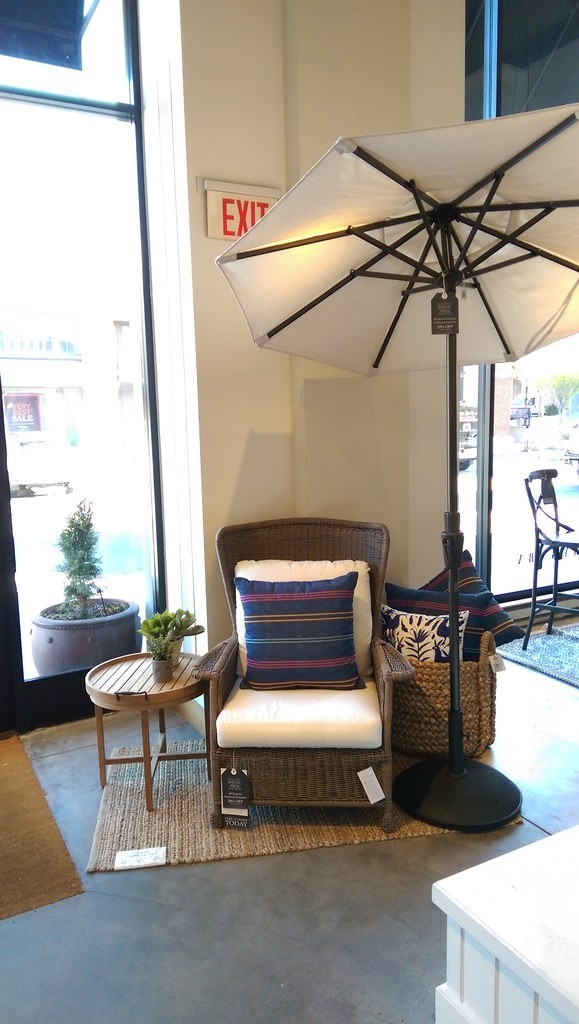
[189,516,415,835]
[522,469,579,650]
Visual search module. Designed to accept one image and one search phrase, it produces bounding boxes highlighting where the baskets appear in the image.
[392,631,497,758]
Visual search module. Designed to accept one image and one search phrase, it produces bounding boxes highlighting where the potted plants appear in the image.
[135,607,206,667]
[145,630,174,683]
[31,499,143,678]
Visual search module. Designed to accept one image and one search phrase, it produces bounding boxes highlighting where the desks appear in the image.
[431,823,579,1024]
[84,652,210,812]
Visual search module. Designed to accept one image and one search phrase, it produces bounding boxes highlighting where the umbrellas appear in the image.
[216,102,579,777]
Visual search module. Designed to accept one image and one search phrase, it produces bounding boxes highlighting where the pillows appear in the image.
[418,549,526,648]
[380,604,470,662]
[232,571,366,691]
[384,583,494,662]
[234,559,374,679]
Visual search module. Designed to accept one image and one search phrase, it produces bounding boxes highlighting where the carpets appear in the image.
[495,622,579,689]
[0,731,87,921]
[85,738,523,873]
